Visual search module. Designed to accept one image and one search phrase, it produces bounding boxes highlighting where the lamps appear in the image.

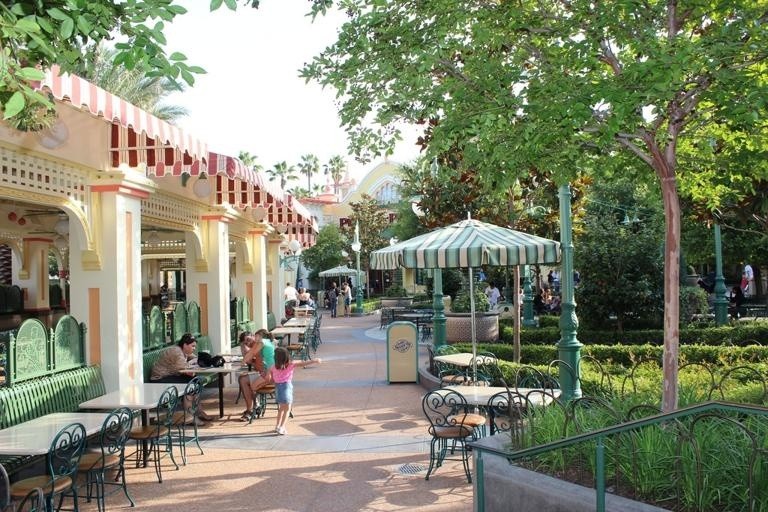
[54,215,69,235]
[289,239,301,252]
[193,173,212,199]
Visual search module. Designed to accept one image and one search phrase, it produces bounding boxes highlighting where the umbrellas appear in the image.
[369,210,563,386]
[318,263,358,289]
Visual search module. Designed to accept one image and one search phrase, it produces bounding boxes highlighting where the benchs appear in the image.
[379,306,435,342]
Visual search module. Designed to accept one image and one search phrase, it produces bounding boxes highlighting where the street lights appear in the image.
[521,199,551,330]
[351,219,367,314]
[408,154,449,371]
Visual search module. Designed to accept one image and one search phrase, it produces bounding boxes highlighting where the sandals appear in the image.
[241,406,261,421]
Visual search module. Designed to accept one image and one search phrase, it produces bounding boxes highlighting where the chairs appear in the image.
[422,348,502,483]
[179,304,322,426]
[0,376,208,512]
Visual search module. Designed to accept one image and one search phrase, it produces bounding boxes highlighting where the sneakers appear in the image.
[274,427,286,435]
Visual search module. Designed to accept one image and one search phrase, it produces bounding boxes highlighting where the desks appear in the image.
[421,386,563,449]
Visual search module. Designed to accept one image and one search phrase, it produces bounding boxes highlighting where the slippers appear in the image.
[185,412,211,426]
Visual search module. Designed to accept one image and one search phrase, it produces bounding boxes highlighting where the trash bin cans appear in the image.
[386,320,420,384]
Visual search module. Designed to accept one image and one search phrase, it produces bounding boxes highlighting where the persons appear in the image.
[255,347,322,436]
[299,287,310,305]
[239,333,277,421]
[285,282,300,310]
[150,333,214,426]
[323,277,352,319]
[729,286,746,312]
[480,267,582,316]
[744,263,754,297]
[307,294,316,313]
[740,272,747,291]
[238,328,276,391]
